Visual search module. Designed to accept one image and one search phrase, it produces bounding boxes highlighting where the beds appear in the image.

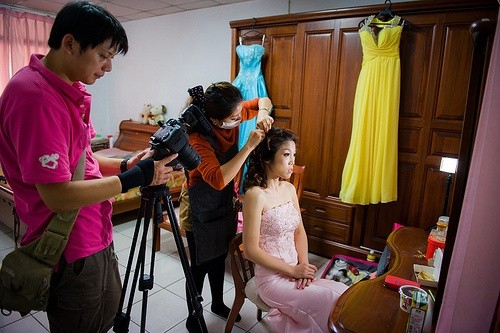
[0,120,184,239]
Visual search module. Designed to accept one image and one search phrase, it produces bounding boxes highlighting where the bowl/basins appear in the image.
[398,285,428,313]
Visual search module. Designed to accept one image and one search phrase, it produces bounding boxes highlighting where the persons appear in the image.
[180,81,274,333]
[240,127,352,333]
[0,0,179,333]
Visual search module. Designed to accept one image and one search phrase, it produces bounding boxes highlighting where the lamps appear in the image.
[440,157,458,217]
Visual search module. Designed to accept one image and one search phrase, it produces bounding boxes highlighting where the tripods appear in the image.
[112,185,210,333]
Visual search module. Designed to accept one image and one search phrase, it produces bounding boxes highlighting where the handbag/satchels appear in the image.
[0,249,52,317]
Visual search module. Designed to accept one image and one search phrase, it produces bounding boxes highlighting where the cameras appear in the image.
[147,104,214,171]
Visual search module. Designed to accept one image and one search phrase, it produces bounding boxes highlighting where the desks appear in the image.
[328,226,428,333]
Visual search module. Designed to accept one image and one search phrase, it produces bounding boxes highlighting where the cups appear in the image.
[436,222,447,232]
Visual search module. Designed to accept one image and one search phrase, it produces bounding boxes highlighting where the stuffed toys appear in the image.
[141,103,167,125]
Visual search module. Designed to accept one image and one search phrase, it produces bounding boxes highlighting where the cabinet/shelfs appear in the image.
[229,0,500,260]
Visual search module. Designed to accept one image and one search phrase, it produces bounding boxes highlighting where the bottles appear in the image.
[366,250,376,262]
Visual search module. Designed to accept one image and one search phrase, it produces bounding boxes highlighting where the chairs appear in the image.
[224,232,263,333]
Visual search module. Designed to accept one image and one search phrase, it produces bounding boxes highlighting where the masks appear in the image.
[218,120,240,129]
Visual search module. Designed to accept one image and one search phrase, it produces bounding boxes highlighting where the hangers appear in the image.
[358,0,412,28]
[236,17,267,41]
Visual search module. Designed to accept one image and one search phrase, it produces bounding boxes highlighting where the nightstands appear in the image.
[91,137,109,153]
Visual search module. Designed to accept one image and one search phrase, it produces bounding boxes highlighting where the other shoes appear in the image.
[211,302,241,323]
[186,314,208,333]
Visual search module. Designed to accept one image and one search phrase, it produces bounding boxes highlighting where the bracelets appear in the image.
[257,108,271,114]
[120,157,130,173]
